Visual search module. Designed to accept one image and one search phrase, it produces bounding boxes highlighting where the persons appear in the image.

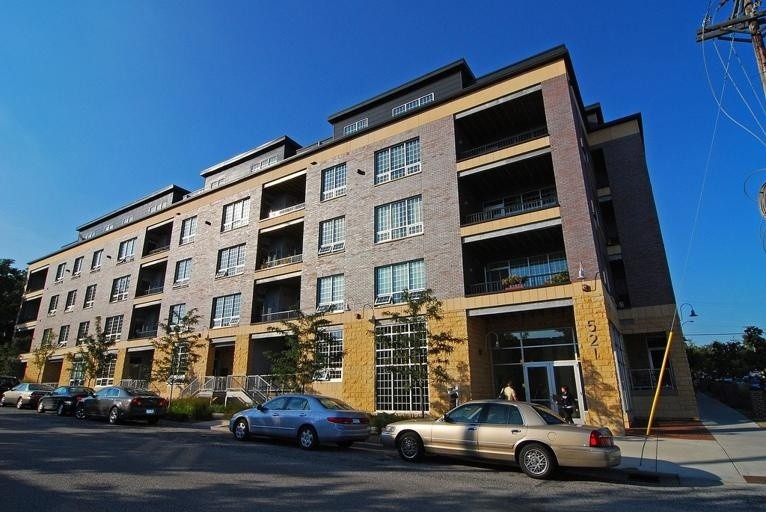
[555,385,578,424]
[499,382,519,401]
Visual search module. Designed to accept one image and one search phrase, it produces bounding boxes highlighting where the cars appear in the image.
[380,398,621,479]
[229,394,371,450]
[74,386,166,424]
[736,378,761,388]
[0,376,96,416]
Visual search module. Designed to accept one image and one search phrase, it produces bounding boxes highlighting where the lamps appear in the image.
[344,294,361,320]
[577,259,591,292]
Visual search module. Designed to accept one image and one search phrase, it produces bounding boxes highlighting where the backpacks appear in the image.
[497,394,505,399]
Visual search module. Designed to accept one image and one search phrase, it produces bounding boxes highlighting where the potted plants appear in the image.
[501,274,530,292]
